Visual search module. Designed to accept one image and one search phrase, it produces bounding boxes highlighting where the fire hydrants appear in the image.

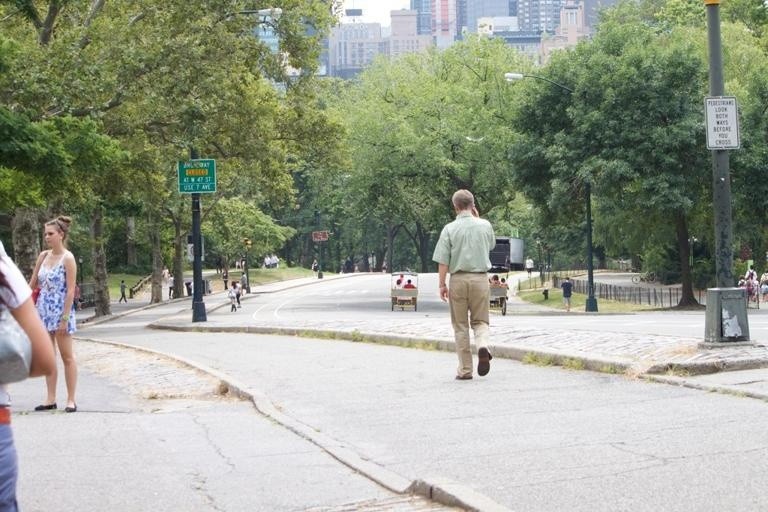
[542,289,548,299]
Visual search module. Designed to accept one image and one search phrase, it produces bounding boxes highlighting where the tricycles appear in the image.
[487,281,509,316]
[391,271,417,311]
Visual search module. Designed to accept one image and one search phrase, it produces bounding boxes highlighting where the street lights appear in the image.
[687,235,698,268]
[239,237,252,293]
[504,72,598,312]
[79,257,84,300]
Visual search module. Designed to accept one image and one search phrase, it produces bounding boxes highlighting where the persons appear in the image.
[26,216,81,415]
[311,259,318,276]
[394,279,402,289]
[498,277,510,290]
[228,280,238,311]
[525,255,534,279]
[542,282,549,301]
[1,241,60,512]
[734,262,768,304]
[236,280,243,308]
[404,280,415,289]
[168,273,175,299]
[491,274,500,284]
[119,279,128,303]
[162,265,170,280]
[559,276,574,313]
[431,188,498,381]
[241,271,248,290]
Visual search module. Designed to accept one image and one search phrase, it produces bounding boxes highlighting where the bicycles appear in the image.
[632,272,656,284]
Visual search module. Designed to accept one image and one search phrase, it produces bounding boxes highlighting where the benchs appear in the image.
[392,287,417,302]
[489,287,506,300]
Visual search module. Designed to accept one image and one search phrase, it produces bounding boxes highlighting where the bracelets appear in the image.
[60,315,71,321]
[439,283,446,288]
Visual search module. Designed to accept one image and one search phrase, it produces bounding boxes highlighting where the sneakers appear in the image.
[65,402,77,412]
[35,402,58,411]
[477,347,491,376]
[455,375,474,380]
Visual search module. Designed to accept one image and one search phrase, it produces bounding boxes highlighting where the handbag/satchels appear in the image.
[32,288,42,305]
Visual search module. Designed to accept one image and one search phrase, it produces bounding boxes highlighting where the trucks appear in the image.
[490,236,524,272]
